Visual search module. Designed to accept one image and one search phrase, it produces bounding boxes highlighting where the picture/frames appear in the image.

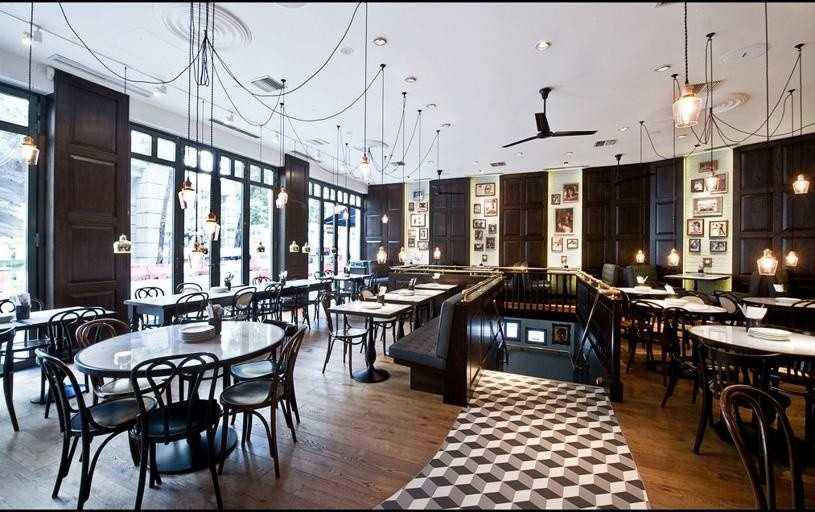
[473,183,498,262]
[552,323,571,346]
[551,181,580,263]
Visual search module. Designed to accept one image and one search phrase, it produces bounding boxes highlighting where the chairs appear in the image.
[76,319,172,405]
[719,384,813,510]
[1,325,21,435]
[221,326,308,477]
[1,272,434,381]
[622,280,814,409]
[131,351,225,509]
[33,347,156,509]
[690,334,791,455]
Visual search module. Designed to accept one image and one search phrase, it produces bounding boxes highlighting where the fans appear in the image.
[501,87,598,149]
[603,155,655,188]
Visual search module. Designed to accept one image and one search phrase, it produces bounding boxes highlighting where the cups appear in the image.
[775,291,785,299]
[376,295,384,306]
[280,278,285,285]
[698,268,704,273]
[14,305,31,319]
[747,317,761,332]
[408,282,415,290]
[225,282,231,290]
[208,317,221,334]
[112,330,132,366]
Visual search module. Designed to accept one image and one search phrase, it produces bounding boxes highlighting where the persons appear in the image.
[556,211,573,233]
[689,240,699,251]
[712,223,725,235]
[564,187,577,202]
[711,242,725,250]
[555,327,568,345]
[691,221,701,233]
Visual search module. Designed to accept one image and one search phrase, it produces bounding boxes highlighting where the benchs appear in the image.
[368,260,784,299]
[387,272,507,407]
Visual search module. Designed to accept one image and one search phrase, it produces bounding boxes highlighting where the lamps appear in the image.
[671,2,701,128]
[783,88,798,268]
[114,66,134,255]
[191,2,208,264]
[382,155,388,225]
[667,72,681,267]
[177,2,198,210]
[397,91,442,259]
[356,4,372,182]
[638,118,645,264]
[792,42,810,194]
[334,124,350,220]
[204,6,220,240]
[288,139,311,254]
[18,1,40,168]
[375,62,389,263]
[757,3,779,274]
[702,32,721,193]
[275,79,287,210]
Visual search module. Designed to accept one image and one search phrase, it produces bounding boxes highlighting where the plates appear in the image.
[424,284,439,287]
[0,314,12,322]
[399,290,414,295]
[635,286,650,292]
[178,325,217,340]
[362,303,380,309]
[748,327,791,340]
[210,286,227,293]
[266,282,280,286]
[775,298,801,302]
[665,298,688,305]
